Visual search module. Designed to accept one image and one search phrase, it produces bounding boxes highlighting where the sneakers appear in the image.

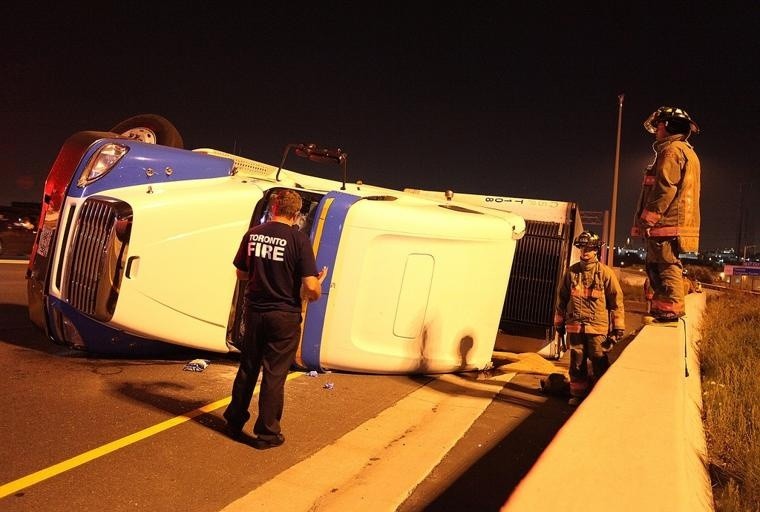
[225,422,285,450]
[642,314,679,327]
[568,396,586,407]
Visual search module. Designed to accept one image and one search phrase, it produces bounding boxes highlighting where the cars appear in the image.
[605,247,742,266]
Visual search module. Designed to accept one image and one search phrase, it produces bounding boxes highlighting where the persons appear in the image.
[555,229,625,404]
[220,186,321,446]
[631,107,702,330]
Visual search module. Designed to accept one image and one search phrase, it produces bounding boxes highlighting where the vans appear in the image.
[23,113,585,378]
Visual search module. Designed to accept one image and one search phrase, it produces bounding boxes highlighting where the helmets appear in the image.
[573,231,601,251]
[643,106,700,135]
